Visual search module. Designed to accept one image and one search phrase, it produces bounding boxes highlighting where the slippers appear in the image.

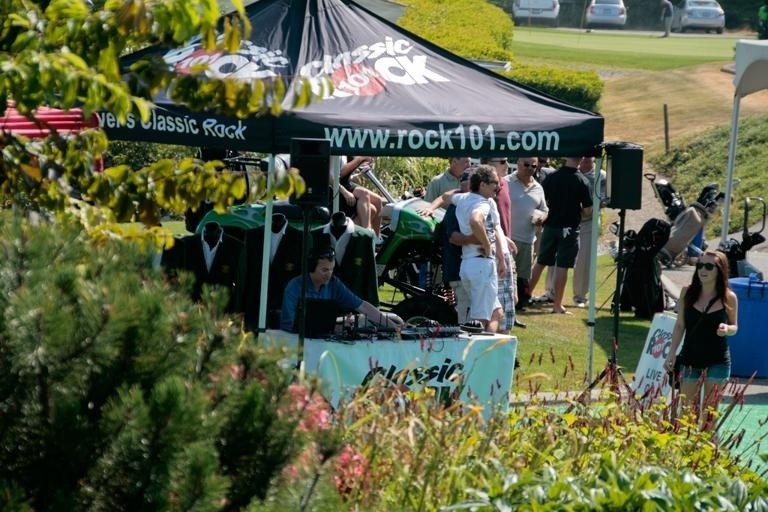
[550,309,576,316]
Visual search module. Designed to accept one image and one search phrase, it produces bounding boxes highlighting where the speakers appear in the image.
[289,155,341,216]
[605,142,643,210]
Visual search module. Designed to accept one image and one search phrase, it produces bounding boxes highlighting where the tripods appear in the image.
[556,209,643,423]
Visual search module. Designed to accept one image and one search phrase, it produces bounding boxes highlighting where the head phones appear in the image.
[306,249,336,273]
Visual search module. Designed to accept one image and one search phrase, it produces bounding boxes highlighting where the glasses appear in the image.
[695,262,717,271]
[519,161,538,168]
[490,159,509,165]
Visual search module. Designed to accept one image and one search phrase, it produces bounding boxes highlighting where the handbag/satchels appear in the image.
[669,354,682,389]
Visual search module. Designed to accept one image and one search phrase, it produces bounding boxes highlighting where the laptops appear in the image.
[293,297,337,335]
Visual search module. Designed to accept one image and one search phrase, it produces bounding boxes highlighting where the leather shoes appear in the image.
[513,318,527,329]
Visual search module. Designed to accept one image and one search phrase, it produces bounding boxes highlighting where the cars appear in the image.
[511,0,560,27]
[586,0,629,30]
[671,0,726,34]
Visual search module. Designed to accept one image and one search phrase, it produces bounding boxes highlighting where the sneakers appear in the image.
[530,293,554,302]
[573,295,588,308]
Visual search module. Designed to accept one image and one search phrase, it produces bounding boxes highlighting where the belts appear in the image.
[475,254,492,258]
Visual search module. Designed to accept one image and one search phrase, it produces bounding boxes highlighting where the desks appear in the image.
[265,326,518,432]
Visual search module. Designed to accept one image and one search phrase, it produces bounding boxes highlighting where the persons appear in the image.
[172,221,241,316]
[504,156,610,315]
[310,210,379,308]
[419,153,527,367]
[269,153,382,236]
[664,250,740,450]
[276,247,403,341]
[244,212,302,329]
